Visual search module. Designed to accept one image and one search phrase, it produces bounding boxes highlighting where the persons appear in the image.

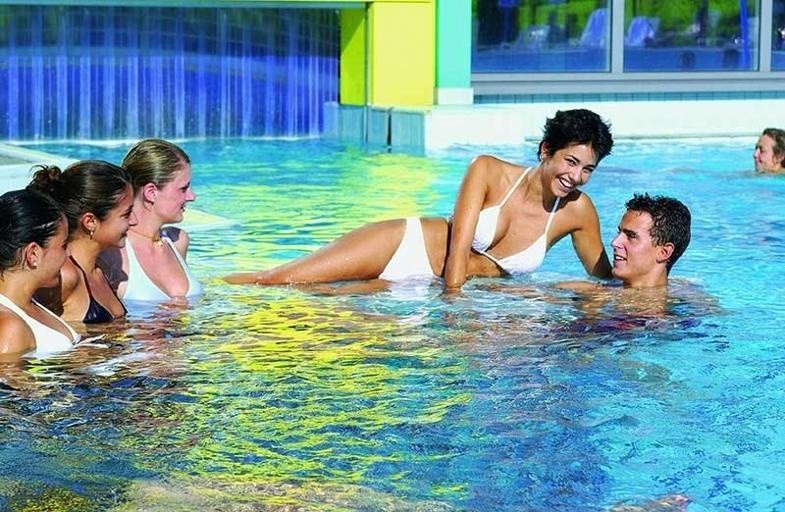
[0,188,83,360]
[548,191,724,323]
[219,108,617,296]
[97,138,204,304]
[24,159,138,324]
[752,126,785,174]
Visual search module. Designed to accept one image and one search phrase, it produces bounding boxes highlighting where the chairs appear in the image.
[498,25,550,47]
[624,17,660,47]
[568,8,607,47]
